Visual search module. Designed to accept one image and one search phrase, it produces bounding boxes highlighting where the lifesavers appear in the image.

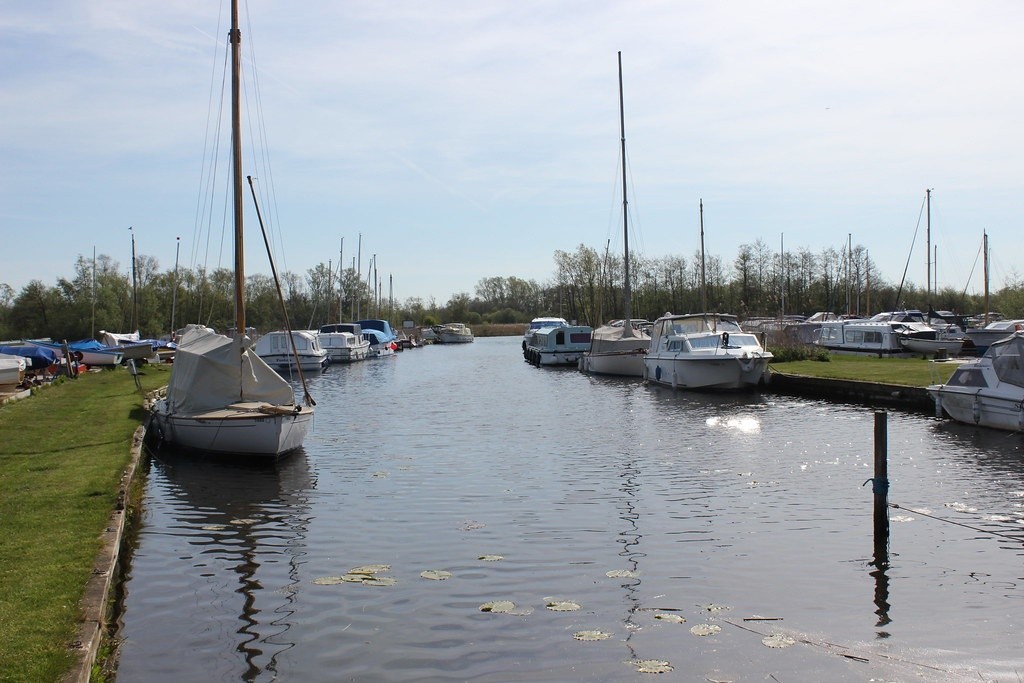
[65,349,84,364]
[522,340,540,366]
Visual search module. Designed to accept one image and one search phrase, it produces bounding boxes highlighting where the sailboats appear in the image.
[142,0,316,457]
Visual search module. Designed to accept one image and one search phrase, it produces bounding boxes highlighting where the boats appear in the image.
[102,330,160,359]
[520,50,1024,434]
[253,230,474,373]
[0,337,125,384]
